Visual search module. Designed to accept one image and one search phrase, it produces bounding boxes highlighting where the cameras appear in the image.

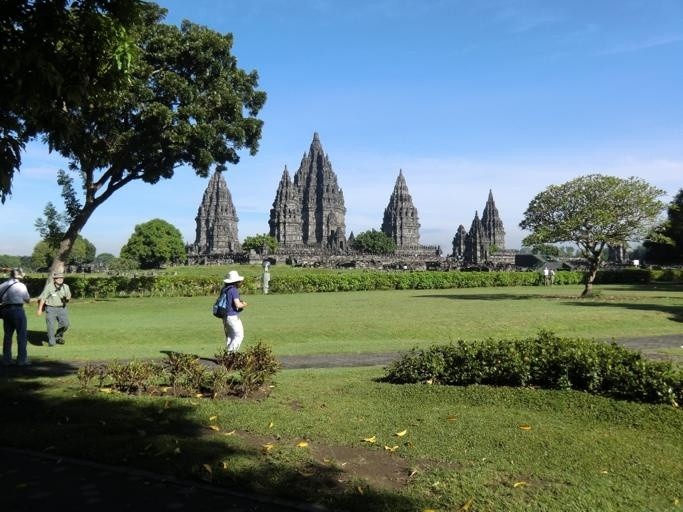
[62,296,69,303]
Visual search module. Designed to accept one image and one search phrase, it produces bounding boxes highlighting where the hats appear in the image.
[224,270,244,284]
[52,271,65,279]
[10,268,26,279]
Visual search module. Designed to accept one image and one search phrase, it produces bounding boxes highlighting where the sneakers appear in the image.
[2,358,31,366]
[48,337,64,347]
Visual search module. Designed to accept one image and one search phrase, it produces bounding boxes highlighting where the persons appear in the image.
[0,268,38,366]
[543,266,555,287]
[213,270,248,355]
[36,271,72,347]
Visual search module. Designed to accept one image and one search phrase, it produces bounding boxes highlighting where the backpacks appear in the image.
[213,285,235,318]
